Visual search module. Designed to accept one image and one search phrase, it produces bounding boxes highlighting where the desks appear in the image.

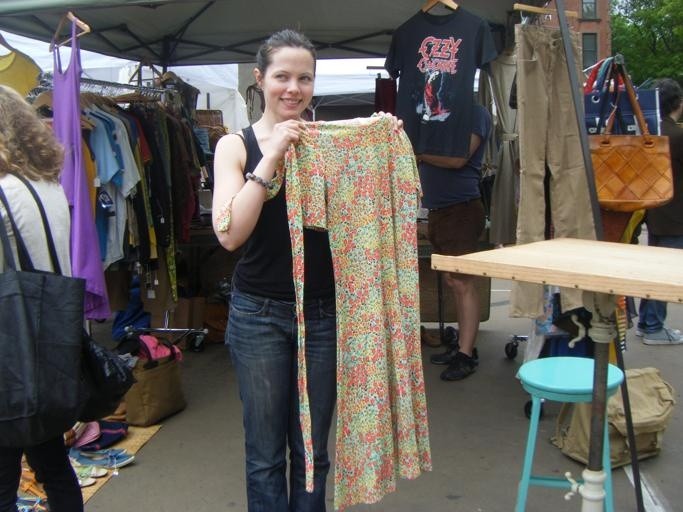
[429,234,681,511]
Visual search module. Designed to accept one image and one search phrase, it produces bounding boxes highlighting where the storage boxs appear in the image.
[534,315,593,359]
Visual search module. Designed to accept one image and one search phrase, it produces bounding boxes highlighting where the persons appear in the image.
[635,77,683,347]
[415,102,493,381]
[210,27,337,512]
[0,83,84,512]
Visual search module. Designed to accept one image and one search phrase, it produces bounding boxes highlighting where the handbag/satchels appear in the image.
[126,353,188,426]
[584,85,674,215]
[77,325,136,425]
[1,269,86,450]
[561,367,675,471]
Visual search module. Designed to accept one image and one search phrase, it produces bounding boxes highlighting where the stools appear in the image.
[512,356,626,512]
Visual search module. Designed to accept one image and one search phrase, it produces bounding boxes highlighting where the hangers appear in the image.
[45,9,94,52]
[0,31,42,72]
[421,0,458,15]
[510,0,579,24]
[30,78,158,113]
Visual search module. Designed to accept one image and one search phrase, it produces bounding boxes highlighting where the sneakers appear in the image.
[431,347,458,365]
[440,350,478,381]
[636,328,683,346]
[69,449,135,486]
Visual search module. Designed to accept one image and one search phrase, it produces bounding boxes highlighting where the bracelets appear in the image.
[245,171,273,190]
